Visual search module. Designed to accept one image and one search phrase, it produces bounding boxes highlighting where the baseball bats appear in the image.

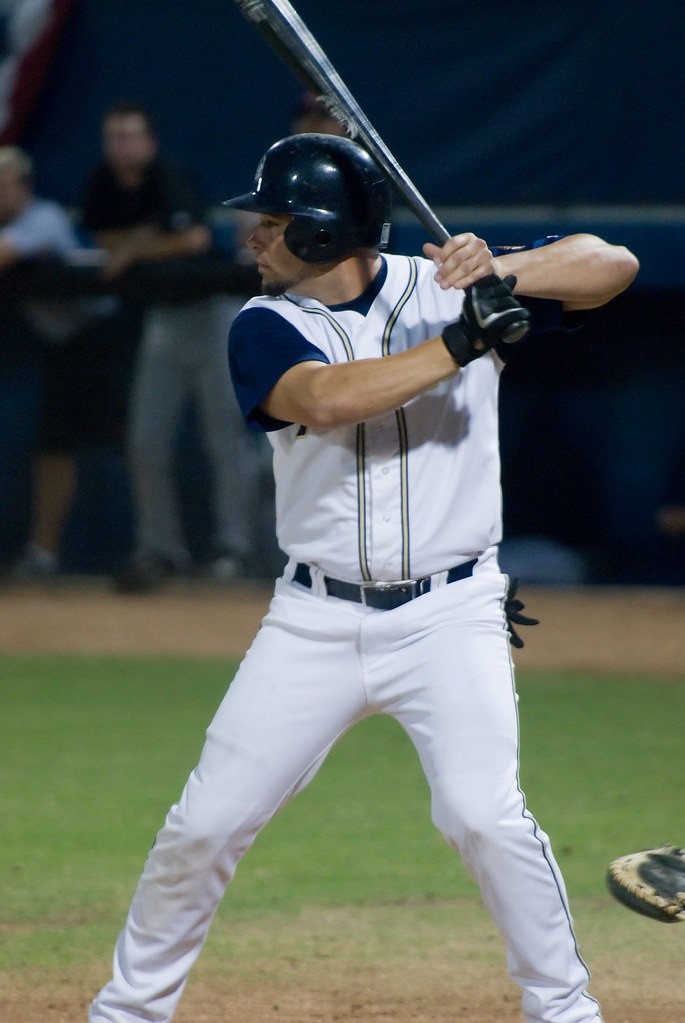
[235,0,530,344]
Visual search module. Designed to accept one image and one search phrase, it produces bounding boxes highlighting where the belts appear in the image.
[294,560,478,610]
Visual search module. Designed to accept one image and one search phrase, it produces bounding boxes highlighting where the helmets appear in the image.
[223,133,389,263]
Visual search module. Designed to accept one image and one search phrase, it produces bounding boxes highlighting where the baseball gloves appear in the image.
[606,843,685,923]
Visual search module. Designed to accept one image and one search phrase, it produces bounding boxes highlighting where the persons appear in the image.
[89,133,640,1023]
[0,94,357,597]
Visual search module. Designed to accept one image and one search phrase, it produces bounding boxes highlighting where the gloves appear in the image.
[442,272,531,368]
